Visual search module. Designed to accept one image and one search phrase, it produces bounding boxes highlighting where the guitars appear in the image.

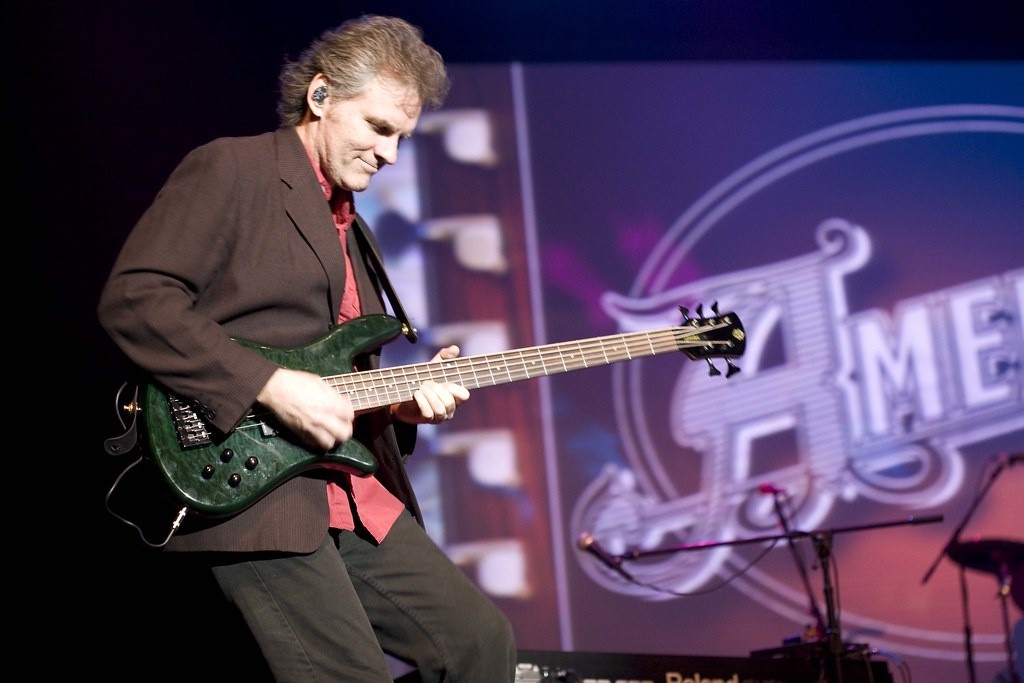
[150,300,746,521]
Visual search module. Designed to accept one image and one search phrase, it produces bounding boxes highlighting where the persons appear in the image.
[99,16,518,683]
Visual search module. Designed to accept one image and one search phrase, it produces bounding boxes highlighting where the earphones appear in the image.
[313,86,329,104]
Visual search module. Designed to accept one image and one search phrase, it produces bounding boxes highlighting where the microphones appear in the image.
[577,531,634,583]
[990,450,1024,464]
